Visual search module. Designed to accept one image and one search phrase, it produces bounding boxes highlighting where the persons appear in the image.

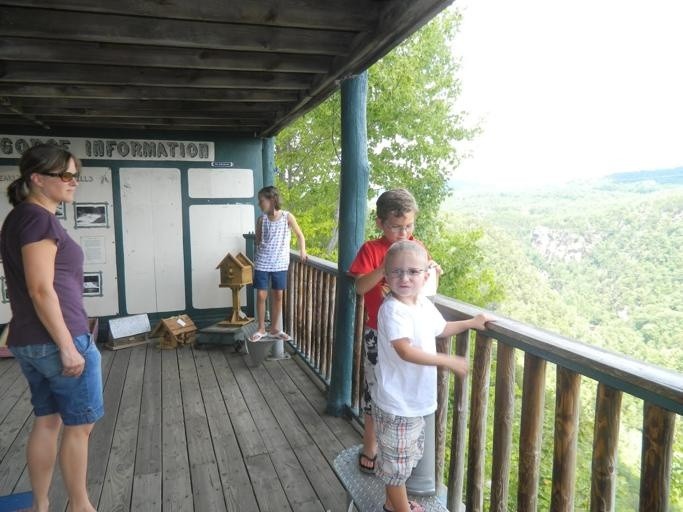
[0,144,106,512]
[248,186,307,343]
[350,189,443,474]
[370,240,497,512]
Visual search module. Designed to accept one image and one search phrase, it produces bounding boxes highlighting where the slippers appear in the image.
[248,332,268,343]
[358,449,378,474]
[268,331,292,341]
[382,500,426,511]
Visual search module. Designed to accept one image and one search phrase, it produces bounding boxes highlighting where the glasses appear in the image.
[40,170,81,183]
[384,221,417,233]
[384,266,431,277]
